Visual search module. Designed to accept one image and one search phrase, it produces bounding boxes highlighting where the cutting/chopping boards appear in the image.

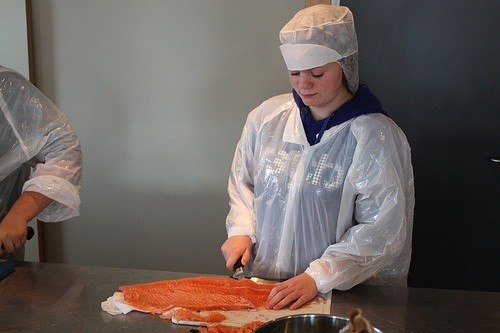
[172,276,332,327]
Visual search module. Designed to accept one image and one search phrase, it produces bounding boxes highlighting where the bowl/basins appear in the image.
[248,314,383,333]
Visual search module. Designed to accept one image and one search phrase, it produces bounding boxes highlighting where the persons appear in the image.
[220,4,414,312]
[0,66,83,263]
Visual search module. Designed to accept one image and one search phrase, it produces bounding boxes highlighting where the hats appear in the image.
[278,3,360,94]
